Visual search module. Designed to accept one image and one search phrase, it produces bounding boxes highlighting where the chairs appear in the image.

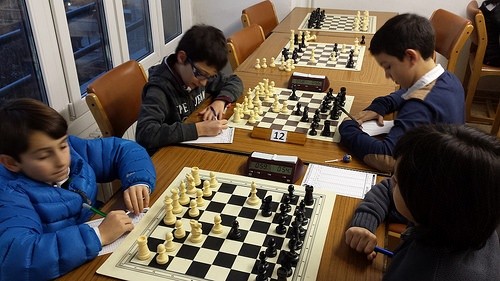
[86,59,148,138]
[225,24,265,72]
[427,9,474,73]
[462,0,500,125]
[241,0,279,39]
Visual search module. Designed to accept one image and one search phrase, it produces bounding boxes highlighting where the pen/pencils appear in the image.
[337,105,363,129]
[209,106,217,120]
[82,202,107,218]
[374,246,394,257]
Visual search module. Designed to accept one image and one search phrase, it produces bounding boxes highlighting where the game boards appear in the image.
[95,167,338,281]
[225,85,355,143]
[298,12,377,34]
[274,40,366,72]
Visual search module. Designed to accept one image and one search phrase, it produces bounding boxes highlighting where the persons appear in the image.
[0,98,156,281]
[470,0,500,68]
[135,24,244,158]
[338,13,465,174]
[343,122,500,281]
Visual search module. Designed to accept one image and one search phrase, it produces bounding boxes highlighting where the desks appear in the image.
[58,6,398,281]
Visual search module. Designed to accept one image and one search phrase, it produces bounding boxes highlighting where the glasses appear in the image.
[186,57,218,82]
